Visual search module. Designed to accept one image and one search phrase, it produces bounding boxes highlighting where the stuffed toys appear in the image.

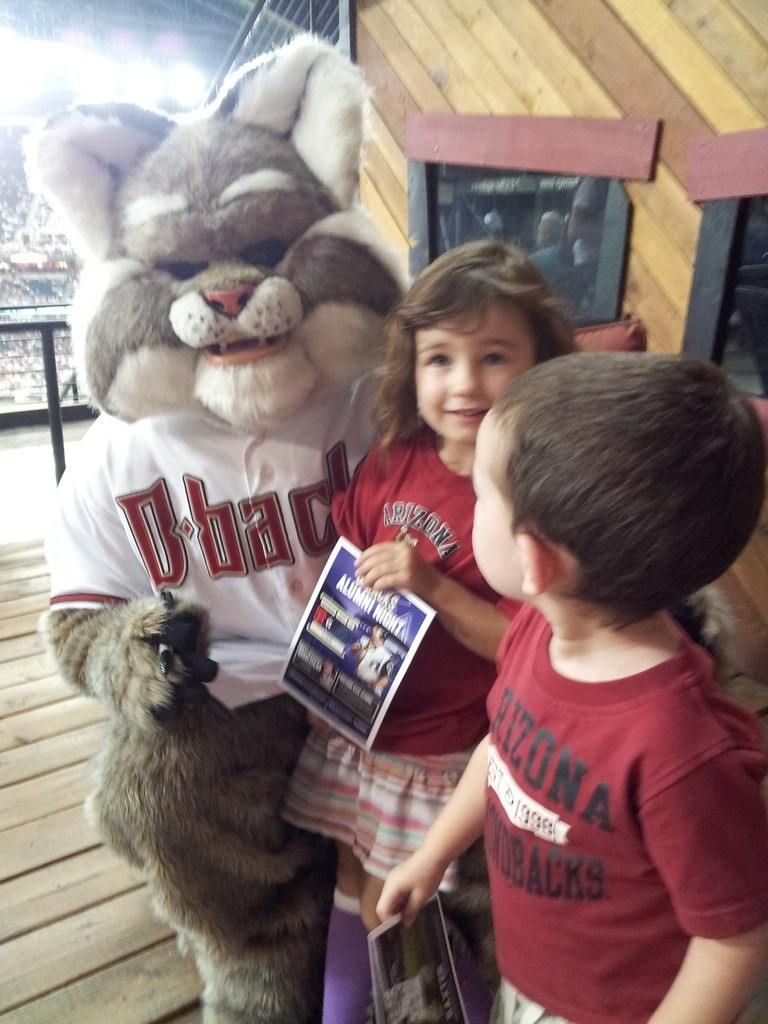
[19,34,730,1024]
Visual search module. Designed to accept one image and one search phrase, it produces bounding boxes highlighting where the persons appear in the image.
[377,352,768,1024]
[280,238,585,1024]
